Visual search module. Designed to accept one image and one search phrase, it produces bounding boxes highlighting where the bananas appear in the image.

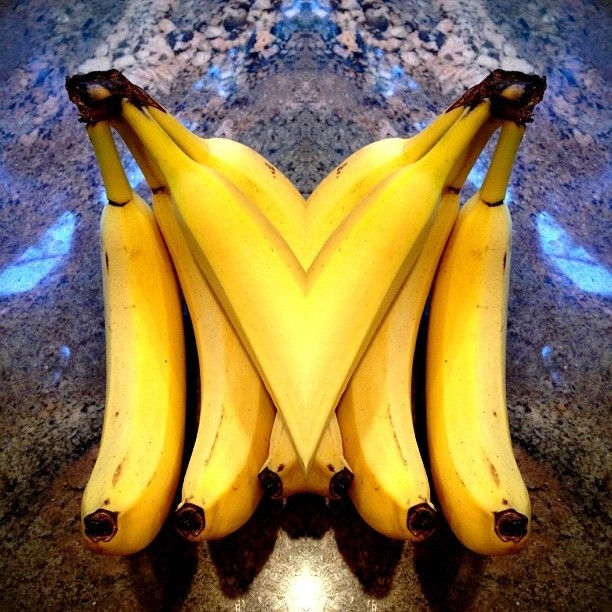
[257,68,549,554]
[63,66,353,554]
